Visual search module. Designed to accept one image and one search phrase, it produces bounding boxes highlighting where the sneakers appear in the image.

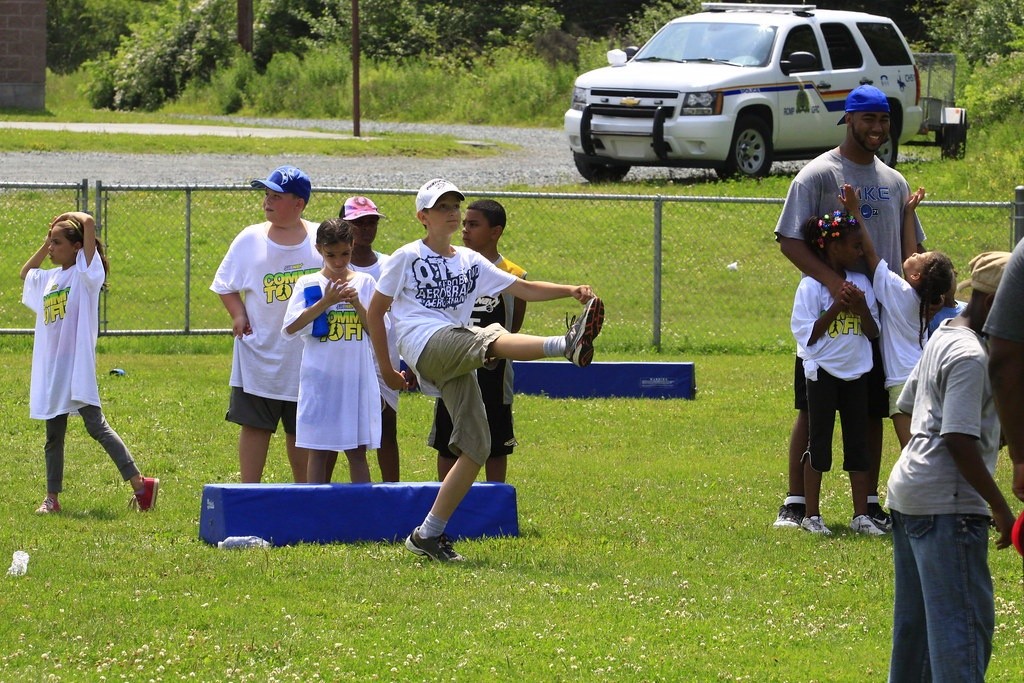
[36,495,61,514]
[564,297,605,368]
[127,478,159,512]
[406,526,464,560]
[868,508,893,531]
[801,515,832,532]
[773,491,806,526]
[849,514,883,535]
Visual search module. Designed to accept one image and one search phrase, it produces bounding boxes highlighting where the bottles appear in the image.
[304,281,329,338]
[217,536,270,550]
[6,550,29,578]
[110,369,124,376]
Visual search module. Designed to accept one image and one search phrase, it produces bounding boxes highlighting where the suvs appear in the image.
[563,2,924,184]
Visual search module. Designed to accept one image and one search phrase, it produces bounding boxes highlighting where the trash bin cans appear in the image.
[941,124,966,160]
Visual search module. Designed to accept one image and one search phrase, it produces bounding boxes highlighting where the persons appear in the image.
[774,83,930,534]
[426,199,528,483]
[929,270,970,340]
[790,211,882,536]
[207,164,324,483]
[982,236,1024,502]
[886,252,1016,683]
[19,211,160,516]
[366,177,605,564]
[338,195,417,482]
[279,217,391,483]
[838,184,953,451]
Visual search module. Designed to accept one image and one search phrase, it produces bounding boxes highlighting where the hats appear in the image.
[837,83,891,125]
[339,196,385,220]
[957,251,1012,296]
[416,179,465,213]
[251,164,311,202]
